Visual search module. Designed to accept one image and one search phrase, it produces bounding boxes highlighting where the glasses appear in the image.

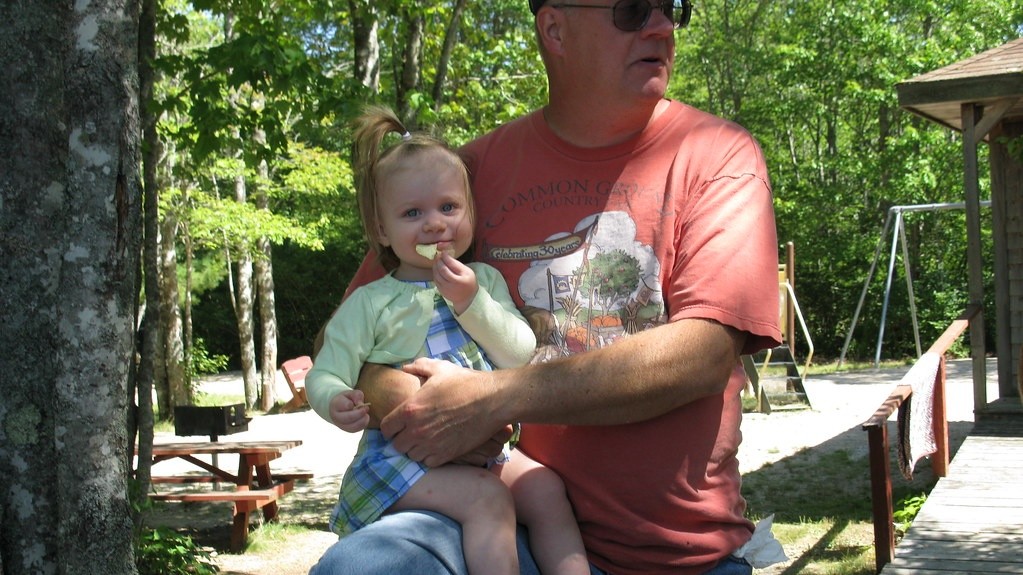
[551,0,694,32]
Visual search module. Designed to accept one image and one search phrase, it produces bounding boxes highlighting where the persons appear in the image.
[310,0,782,575]
[305,107,592,575]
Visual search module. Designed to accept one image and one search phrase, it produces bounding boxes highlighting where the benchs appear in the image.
[145,490,274,546]
[282,355,313,407]
[150,472,314,522]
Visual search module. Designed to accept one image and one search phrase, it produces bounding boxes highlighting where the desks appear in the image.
[133,440,303,545]
